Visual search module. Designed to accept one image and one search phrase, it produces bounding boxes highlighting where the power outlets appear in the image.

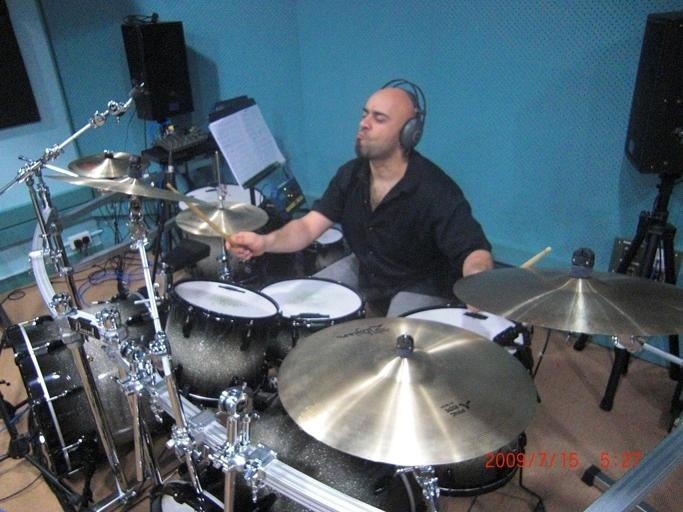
[67,231,92,251]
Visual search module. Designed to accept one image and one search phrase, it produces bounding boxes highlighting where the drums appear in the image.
[398,306,532,498]
[176,183,274,286]
[258,275,366,393]
[164,278,279,408]
[8,286,167,482]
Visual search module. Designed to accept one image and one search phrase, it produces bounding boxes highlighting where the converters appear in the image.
[74,240,81,248]
[83,237,89,243]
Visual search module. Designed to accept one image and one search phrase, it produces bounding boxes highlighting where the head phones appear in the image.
[382,79,426,149]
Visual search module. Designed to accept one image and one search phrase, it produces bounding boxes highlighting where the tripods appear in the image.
[574,175,683,411]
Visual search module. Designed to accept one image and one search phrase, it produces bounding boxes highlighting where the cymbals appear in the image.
[176,202,270,236]
[453,267,683,337]
[278,317,537,467]
[43,174,209,207]
[68,152,150,178]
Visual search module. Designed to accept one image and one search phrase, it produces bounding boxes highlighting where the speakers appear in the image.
[121,21,193,120]
[625,12,683,173]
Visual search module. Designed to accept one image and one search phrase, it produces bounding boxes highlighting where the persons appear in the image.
[221,77,497,325]
